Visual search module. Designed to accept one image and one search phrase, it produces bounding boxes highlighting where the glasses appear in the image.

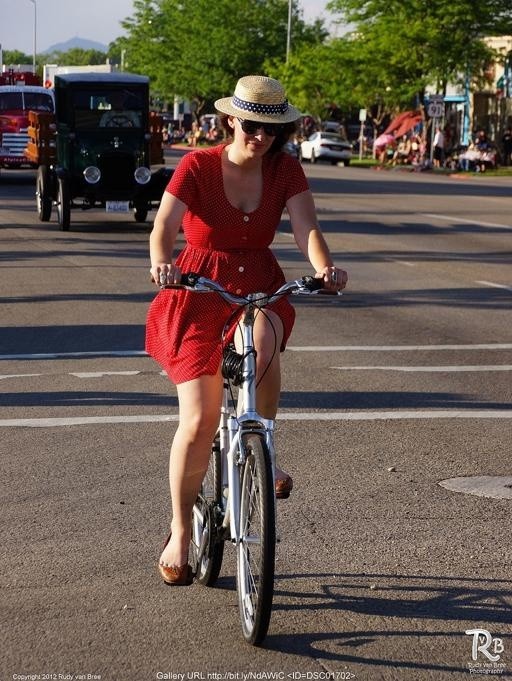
[236,118,284,136]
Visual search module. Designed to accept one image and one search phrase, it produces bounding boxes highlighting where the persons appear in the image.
[147,74,349,584]
[374,127,502,175]
[152,109,220,146]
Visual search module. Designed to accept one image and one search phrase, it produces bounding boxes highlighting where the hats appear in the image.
[213,75,303,123]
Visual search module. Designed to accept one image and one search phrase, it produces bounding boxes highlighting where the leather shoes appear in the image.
[158,530,193,583]
[274,473,293,493]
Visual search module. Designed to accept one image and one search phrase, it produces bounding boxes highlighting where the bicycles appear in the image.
[150,273,343,645]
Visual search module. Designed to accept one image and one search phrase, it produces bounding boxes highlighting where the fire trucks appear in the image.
[0,71,56,174]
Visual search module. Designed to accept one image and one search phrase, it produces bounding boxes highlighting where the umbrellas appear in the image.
[383,111,415,134]
[393,115,423,140]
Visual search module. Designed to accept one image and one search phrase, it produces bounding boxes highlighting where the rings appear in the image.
[175,274,182,279]
[158,272,168,287]
[332,272,337,282]
[166,273,173,278]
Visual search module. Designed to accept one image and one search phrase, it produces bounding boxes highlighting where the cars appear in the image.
[299,131,352,166]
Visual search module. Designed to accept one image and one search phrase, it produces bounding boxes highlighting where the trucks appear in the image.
[26,72,176,230]
